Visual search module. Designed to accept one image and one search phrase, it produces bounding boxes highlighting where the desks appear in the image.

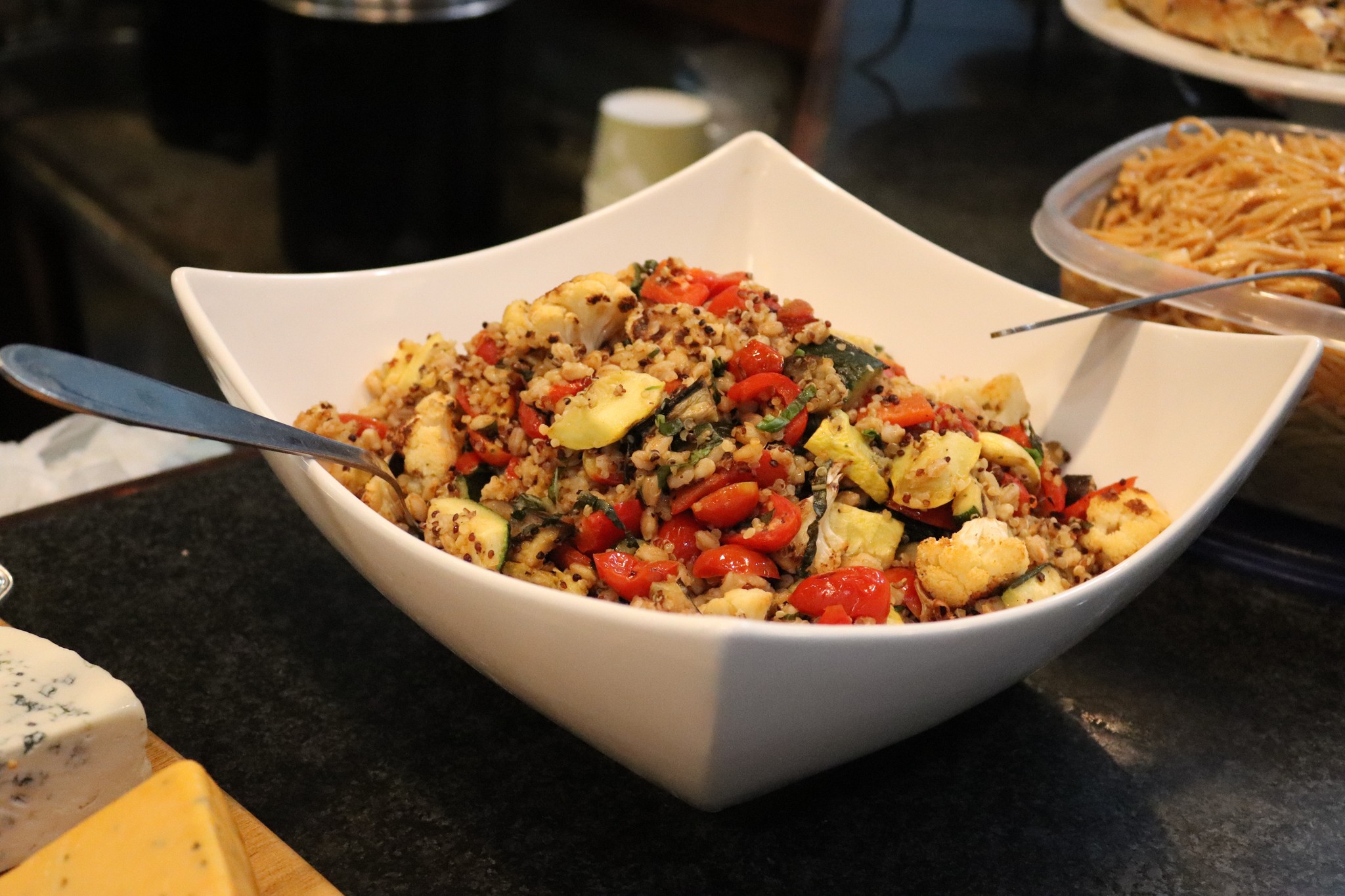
[0,446,1345,896]
[0,105,288,445]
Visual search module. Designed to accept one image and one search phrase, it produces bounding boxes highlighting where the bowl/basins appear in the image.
[1030,117,1345,448]
[165,130,1323,813]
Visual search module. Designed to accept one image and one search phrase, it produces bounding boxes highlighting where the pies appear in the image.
[1117,0,1345,75]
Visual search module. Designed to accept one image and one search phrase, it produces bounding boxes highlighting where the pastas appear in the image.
[1056,114,1344,447]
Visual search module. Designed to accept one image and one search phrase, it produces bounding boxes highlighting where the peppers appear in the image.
[336,255,1139,625]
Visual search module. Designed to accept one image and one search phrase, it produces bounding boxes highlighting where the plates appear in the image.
[1061,0,1345,106]
